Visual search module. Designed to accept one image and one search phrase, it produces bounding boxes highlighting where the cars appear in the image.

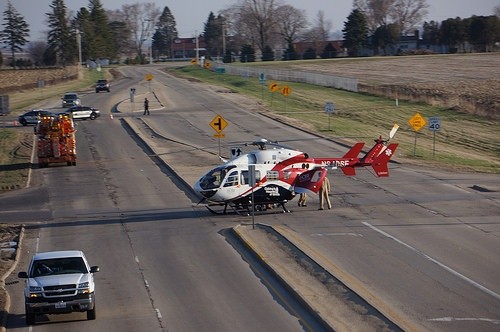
[60,92,79,107]
[18,109,58,126]
[95,78,112,93]
[55,106,101,121]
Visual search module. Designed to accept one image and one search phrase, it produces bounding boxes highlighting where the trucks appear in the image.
[34,116,78,168]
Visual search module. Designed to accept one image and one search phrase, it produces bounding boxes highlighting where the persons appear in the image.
[298,193,307,207]
[143,98,150,115]
[317,172,332,210]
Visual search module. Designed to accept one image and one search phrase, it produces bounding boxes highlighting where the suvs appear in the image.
[18,250,100,326]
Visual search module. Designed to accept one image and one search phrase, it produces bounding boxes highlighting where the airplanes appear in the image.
[192,135,399,217]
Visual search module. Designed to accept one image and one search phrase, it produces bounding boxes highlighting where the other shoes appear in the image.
[297,202,301,207]
[328,208,331,209]
[302,205,307,206]
[318,208,323,210]
